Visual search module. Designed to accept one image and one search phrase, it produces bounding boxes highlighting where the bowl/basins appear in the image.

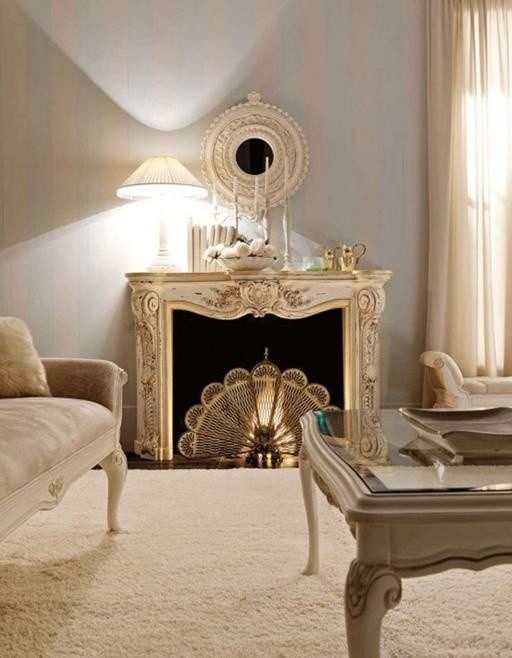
[216,257,279,272]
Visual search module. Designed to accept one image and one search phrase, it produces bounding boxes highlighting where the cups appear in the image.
[303,256,327,269]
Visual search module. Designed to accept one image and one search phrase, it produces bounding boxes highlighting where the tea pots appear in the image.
[319,244,344,272]
[338,242,366,271]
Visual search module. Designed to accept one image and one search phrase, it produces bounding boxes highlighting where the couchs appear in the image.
[417,350,511,409]
[0,316,133,553]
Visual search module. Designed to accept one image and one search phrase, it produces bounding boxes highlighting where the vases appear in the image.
[216,254,281,269]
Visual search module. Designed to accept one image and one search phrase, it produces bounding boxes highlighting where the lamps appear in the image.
[115,154,211,268]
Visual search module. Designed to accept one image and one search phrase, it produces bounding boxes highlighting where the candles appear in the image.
[253,174,260,211]
[211,175,220,214]
[231,174,239,203]
[264,156,270,204]
[282,156,289,202]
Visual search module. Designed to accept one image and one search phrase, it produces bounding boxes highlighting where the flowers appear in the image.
[202,231,286,265]
